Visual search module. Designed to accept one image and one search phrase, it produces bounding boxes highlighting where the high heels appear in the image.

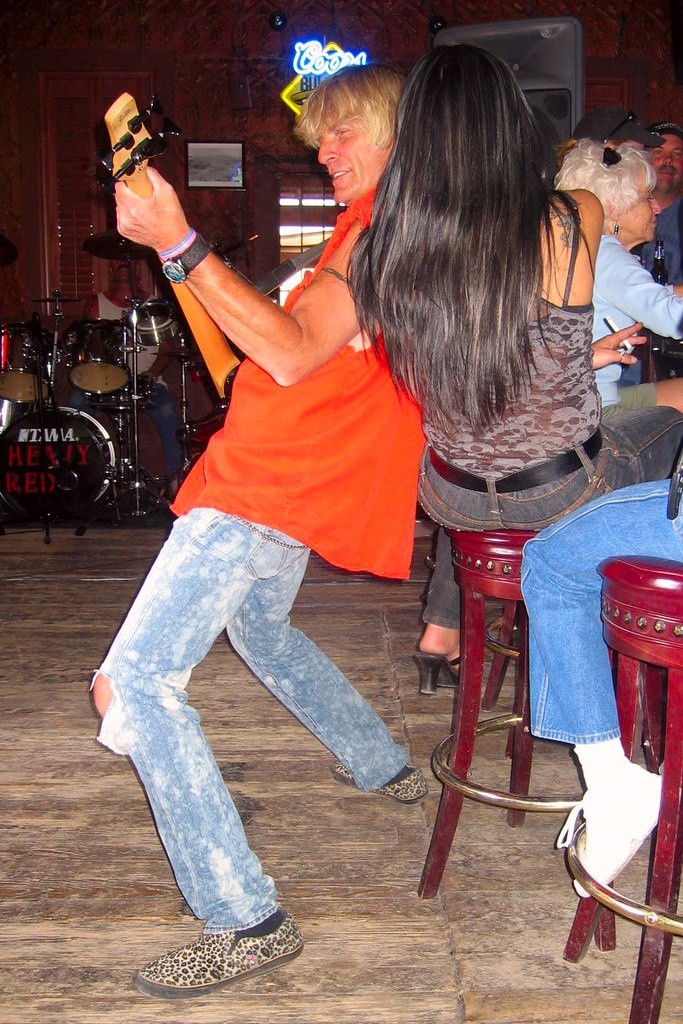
[414,651,460,694]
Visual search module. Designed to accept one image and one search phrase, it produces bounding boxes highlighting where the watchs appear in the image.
[162,231,211,283]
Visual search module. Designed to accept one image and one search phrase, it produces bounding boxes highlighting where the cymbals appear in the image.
[83,231,154,260]
[0,234,18,267]
[31,297,80,303]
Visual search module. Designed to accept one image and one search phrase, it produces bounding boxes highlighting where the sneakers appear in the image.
[330,759,429,806]
[136,911,304,999]
[556,762,660,897]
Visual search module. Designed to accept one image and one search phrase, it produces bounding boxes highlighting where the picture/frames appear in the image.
[183,138,247,192]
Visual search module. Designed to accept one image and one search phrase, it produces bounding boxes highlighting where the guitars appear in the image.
[96,90,242,465]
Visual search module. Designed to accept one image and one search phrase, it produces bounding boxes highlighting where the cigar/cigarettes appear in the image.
[606,316,631,349]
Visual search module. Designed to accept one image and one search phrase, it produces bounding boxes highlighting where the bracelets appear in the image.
[158,228,196,260]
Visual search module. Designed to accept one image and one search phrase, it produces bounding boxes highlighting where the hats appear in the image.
[645,121,683,141]
[572,108,667,147]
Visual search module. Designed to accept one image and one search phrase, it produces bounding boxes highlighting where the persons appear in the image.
[68,259,182,495]
[553,106,683,408]
[93,65,429,999]
[349,45,683,694]
[520,479,683,897]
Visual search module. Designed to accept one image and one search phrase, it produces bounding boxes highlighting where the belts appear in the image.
[430,427,602,493]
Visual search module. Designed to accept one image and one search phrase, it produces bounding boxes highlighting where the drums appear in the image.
[0,301,180,525]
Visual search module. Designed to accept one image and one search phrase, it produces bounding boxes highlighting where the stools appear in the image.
[562,555,683,1024]
[417,530,616,952]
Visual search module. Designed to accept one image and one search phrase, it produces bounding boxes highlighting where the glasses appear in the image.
[603,111,638,143]
[602,146,621,168]
